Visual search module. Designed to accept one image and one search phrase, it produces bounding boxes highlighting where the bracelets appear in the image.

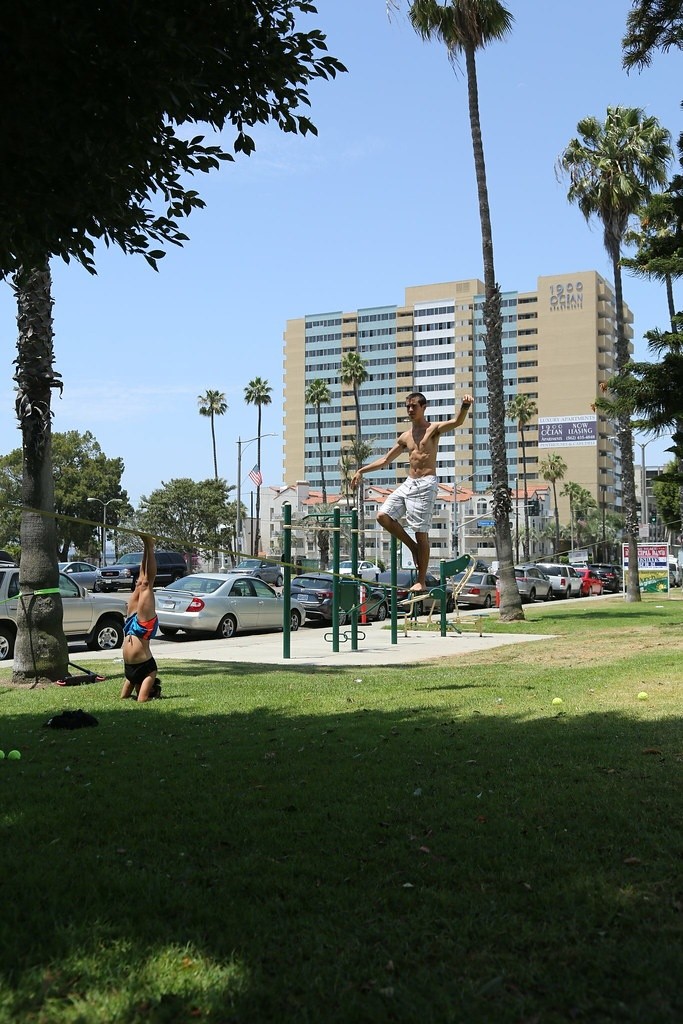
[462,403,471,410]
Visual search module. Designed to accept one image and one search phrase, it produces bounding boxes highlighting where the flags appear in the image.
[249,464,262,486]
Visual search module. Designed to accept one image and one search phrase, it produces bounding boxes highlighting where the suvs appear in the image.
[588,564,623,593]
[535,563,583,599]
[0,561,128,660]
[0,551,14,569]
[231,560,283,587]
[571,562,588,570]
[282,572,389,625]
[496,565,553,602]
[95,552,187,593]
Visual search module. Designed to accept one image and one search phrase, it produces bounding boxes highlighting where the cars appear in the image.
[575,569,603,596]
[371,569,453,615]
[58,562,100,593]
[325,561,381,583]
[154,573,306,639]
[448,571,499,608]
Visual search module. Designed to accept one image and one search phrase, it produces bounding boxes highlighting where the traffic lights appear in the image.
[529,501,540,517]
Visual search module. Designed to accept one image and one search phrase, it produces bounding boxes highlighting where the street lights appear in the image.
[648,518,656,542]
[236,433,279,566]
[607,434,674,542]
[648,509,657,542]
[452,467,534,560]
[87,498,122,565]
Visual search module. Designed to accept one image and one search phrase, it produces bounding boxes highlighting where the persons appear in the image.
[351,393,474,591]
[120,536,161,702]
